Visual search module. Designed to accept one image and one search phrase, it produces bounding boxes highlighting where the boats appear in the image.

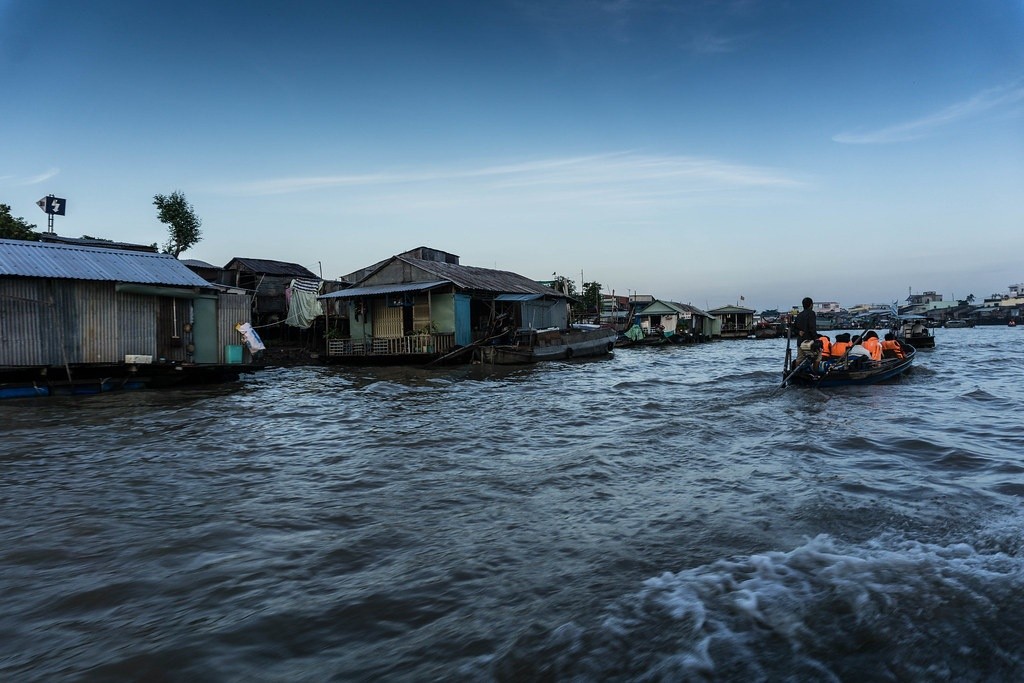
[786,333,918,389]
[890,314,935,348]
[945,319,967,328]
[754,318,777,339]
[471,324,618,367]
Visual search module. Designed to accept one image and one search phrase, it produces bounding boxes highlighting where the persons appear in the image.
[793,297,821,380]
[899,320,912,338]
[912,320,924,337]
[818,331,907,368]
[1009,320,1015,325]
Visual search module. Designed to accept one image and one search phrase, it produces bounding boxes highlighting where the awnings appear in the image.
[317,281,451,300]
[493,294,544,300]
